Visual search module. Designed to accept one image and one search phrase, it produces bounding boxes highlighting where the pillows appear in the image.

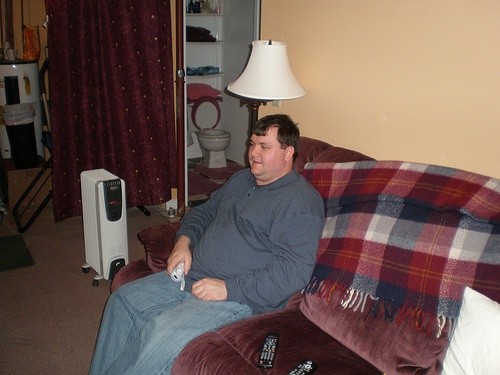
[438,286,500,374]
[301,271,462,374]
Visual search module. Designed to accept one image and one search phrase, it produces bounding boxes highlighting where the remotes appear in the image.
[258,332,279,368]
[288,359,318,375]
[170,263,185,282]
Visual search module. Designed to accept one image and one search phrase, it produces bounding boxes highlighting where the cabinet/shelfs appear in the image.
[182,1,226,106]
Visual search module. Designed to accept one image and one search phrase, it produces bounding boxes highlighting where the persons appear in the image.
[87,115,327,375]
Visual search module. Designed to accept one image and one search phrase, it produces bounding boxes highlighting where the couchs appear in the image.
[109,135,500,375]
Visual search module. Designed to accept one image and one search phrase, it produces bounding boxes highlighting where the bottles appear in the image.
[3,40,10,60]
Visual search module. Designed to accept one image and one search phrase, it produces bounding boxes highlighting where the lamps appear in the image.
[228,41,306,141]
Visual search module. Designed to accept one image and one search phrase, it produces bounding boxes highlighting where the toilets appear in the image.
[194,125,233,169]
